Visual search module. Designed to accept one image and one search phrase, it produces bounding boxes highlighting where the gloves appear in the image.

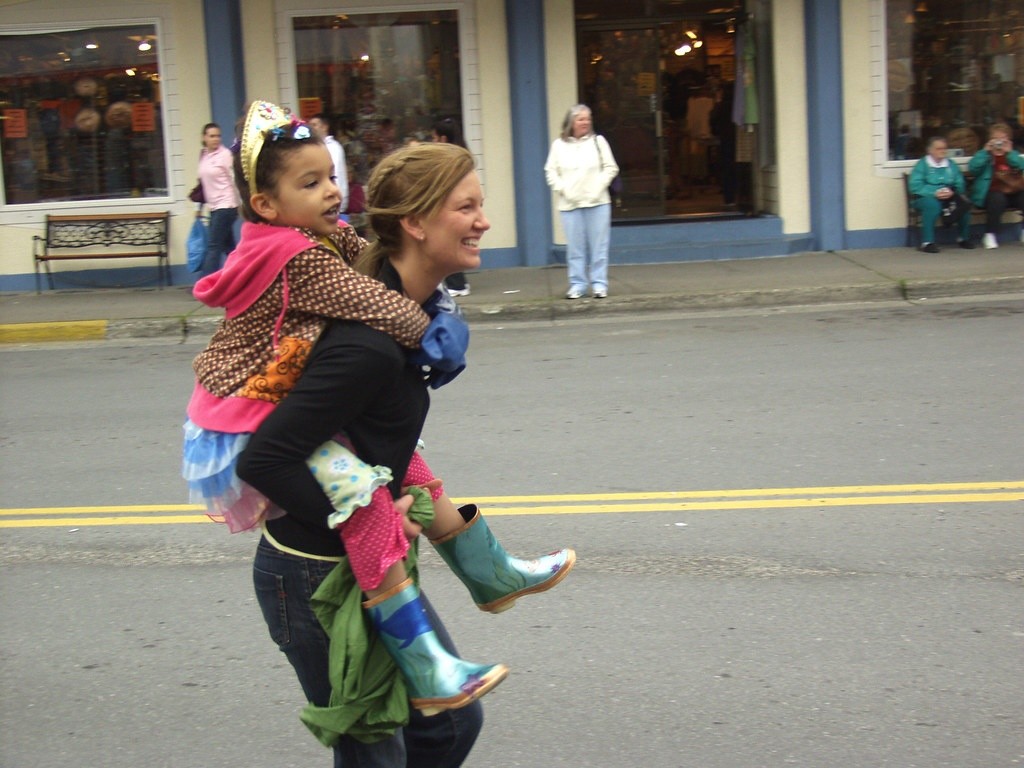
[419,312,470,373]
[407,349,466,390]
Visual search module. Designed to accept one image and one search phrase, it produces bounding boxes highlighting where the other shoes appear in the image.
[980,234,999,249]
[920,241,939,252]
[591,283,609,297]
[957,238,974,248]
[564,285,588,298]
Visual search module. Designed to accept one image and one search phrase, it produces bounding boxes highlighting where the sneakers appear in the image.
[448,282,471,297]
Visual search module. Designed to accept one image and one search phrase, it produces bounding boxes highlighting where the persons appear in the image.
[310,112,349,213]
[194,122,241,278]
[968,123,1024,249]
[430,123,470,297]
[234,141,490,768]
[661,72,688,203]
[709,80,752,207]
[183,98,577,717]
[544,104,620,299]
[907,136,976,253]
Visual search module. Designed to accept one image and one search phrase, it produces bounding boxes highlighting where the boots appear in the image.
[362,575,512,716]
[428,504,577,614]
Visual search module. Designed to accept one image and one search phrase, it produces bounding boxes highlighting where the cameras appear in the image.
[995,141,1005,149]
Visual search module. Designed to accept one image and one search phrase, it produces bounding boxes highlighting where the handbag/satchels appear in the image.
[594,134,624,198]
[184,216,208,273]
[941,187,970,228]
[188,180,204,204]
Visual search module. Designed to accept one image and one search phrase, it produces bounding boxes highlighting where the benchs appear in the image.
[903,170,1024,248]
[32,210,173,293]
